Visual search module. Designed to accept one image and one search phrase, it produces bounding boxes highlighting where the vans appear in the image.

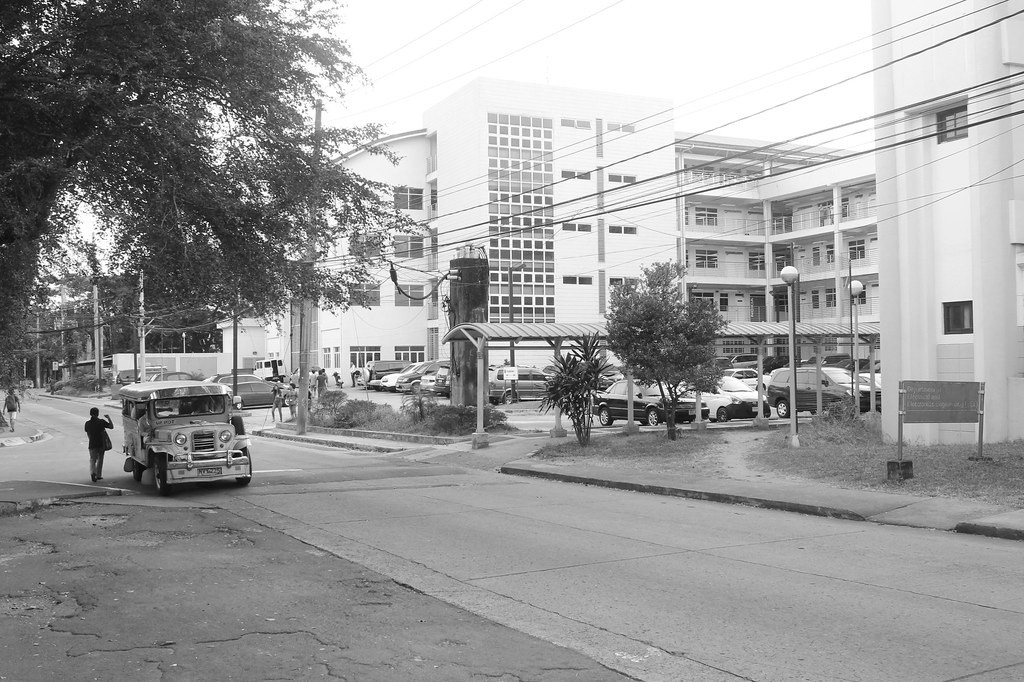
[251,358,286,383]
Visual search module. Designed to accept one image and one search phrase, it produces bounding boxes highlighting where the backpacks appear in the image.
[6,395,16,411]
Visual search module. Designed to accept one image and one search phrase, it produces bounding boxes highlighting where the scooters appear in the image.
[331,372,344,389]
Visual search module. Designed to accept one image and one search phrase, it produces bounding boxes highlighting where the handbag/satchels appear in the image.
[101,419,112,450]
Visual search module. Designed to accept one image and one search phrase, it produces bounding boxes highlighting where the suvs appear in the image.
[118,380,254,491]
[766,365,880,418]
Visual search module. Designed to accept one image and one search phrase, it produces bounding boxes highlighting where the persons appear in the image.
[271,385,284,422]
[84,408,114,481]
[3,388,20,432]
[350,360,357,387]
[49,377,56,390]
[309,370,317,391]
[308,390,312,411]
[322,369,328,389]
[286,383,298,418]
[316,370,326,397]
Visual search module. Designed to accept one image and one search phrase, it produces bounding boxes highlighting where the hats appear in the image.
[8,388,14,393]
[290,383,296,389]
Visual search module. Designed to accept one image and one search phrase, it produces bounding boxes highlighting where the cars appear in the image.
[355,353,626,405]
[109,366,298,410]
[715,351,768,392]
[288,365,322,389]
[590,378,710,427]
[675,375,772,423]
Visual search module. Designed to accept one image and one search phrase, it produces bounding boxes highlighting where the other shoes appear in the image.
[92,475,97,482]
[96,476,103,480]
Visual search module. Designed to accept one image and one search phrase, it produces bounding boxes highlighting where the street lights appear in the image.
[779,266,801,447]
[182,332,186,353]
[847,279,864,419]
[507,264,527,404]
